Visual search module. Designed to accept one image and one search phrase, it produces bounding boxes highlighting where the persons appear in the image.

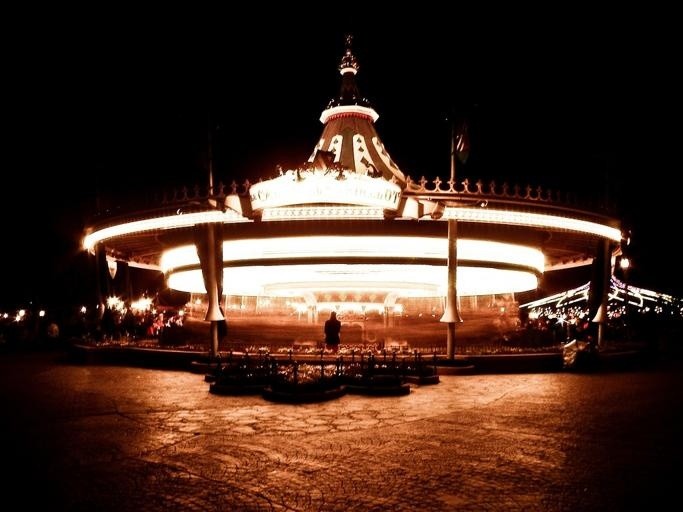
[99,304,183,340]
[324,311,341,354]
[209,307,227,350]
